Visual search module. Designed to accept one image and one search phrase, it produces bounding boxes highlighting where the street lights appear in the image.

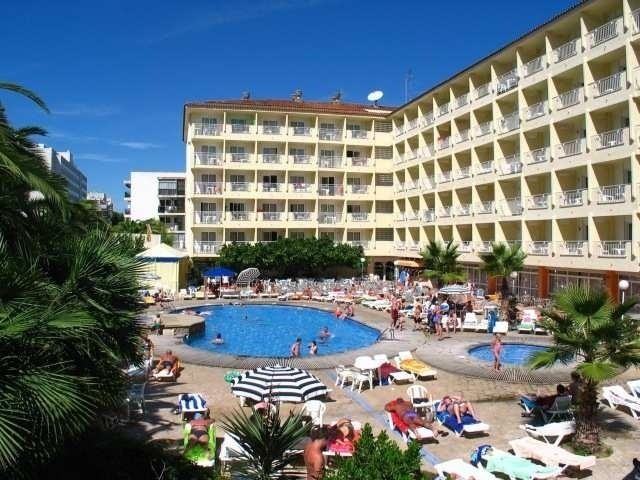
[359,257,366,281]
[617,279,630,342]
[509,270,518,297]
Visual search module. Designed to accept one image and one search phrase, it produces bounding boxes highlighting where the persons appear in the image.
[564,370,584,395]
[383,397,439,442]
[366,269,562,374]
[154,314,161,334]
[213,332,225,344]
[436,390,481,424]
[140,274,362,321]
[152,349,178,377]
[518,383,567,412]
[246,408,356,480]
[290,336,301,356]
[306,340,318,357]
[318,324,332,341]
[181,412,217,459]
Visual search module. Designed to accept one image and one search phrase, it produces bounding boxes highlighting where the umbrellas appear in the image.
[201,265,239,298]
[229,363,328,403]
[235,268,261,298]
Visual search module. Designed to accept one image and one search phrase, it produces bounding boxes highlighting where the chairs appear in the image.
[373,350,437,385]
[406,385,434,413]
[434,398,488,437]
[384,400,433,443]
[463,312,509,335]
[177,392,217,468]
[600,379,640,419]
[335,356,385,393]
[219,432,254,476]
[517,309,550,334]
[521,395,573,424]
[433,436,597,480]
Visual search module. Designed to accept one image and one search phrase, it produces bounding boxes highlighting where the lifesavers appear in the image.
[224,370,241,382]
